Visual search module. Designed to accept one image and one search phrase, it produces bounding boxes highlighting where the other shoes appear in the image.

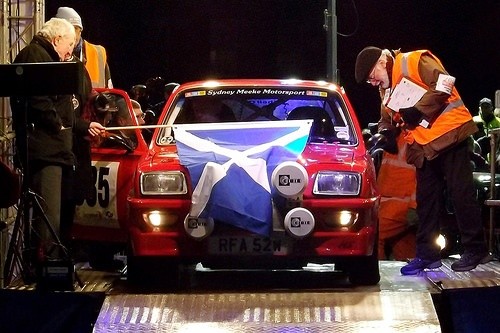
[400,254,442,275]
[452,249,490,271]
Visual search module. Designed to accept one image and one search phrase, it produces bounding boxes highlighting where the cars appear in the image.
[72,78,398,288]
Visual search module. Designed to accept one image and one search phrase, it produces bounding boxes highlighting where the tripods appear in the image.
[5,95,84,291]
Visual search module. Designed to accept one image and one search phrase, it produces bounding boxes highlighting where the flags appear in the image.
[171,118,315,238]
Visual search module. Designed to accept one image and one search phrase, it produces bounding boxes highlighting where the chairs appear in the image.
[188,100,236,122]
[286,105,336,139]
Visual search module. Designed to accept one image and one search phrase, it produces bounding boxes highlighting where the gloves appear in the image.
[399,106,422,129]
[379,129,393,150]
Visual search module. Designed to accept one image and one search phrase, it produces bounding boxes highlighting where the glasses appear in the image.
[137,114,146,119]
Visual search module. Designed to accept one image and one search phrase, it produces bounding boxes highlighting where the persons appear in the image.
[191,96,222,123]
[354,45,492,276]
[52,2,182,263]
[473,97,500,176]
[0,159,21,208]
[9,18,106,265]
[373,122,418,262]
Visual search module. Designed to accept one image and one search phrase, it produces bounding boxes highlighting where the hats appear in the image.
[56,7,83,30]
[479,97,493,106]
[355,46,382,83]
